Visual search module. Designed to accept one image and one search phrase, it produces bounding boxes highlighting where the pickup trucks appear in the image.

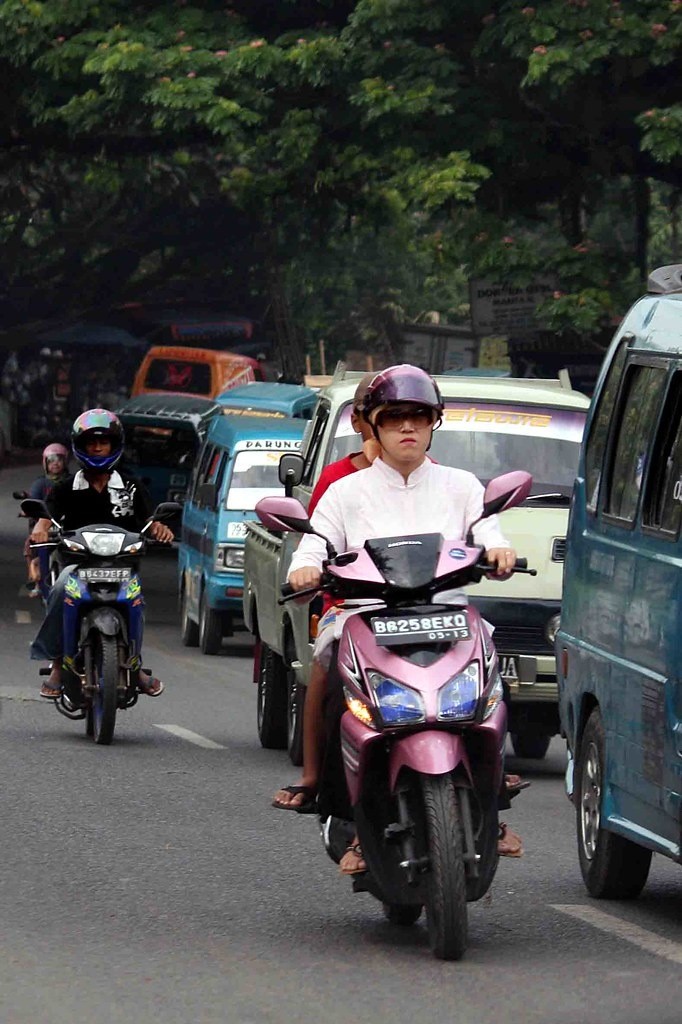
[244,362,595,768]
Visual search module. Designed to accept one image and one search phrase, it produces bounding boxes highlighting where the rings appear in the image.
[506,552,511,554]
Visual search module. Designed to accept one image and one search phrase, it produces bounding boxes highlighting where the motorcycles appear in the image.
[10,489,185,746]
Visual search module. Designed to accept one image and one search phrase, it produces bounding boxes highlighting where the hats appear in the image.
[353,370,381,411]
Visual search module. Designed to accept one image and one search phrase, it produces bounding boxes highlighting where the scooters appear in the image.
[253,470,537,962]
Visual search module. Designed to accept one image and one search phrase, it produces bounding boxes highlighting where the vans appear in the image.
[554,266,682,904]
[109,345,333,657]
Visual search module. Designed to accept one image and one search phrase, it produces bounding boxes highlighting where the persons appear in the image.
[271,372,530,809]
[283,364,523,873]
[22,443,71,597]
[33,408,175,697]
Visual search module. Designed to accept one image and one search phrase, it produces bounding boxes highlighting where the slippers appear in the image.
[342,844,367,875]
[30,590,42,598]
[507,780,531,791]
[271,786,316,810]
[497,835,524,858]
[39,681,61,698]
[140,676,163,696]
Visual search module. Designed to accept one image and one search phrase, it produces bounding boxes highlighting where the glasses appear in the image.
[375,406,434,430]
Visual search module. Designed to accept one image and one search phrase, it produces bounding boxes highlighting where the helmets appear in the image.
[71,409,125,473]
[43,443,70,471]
[362,365,443,422]
